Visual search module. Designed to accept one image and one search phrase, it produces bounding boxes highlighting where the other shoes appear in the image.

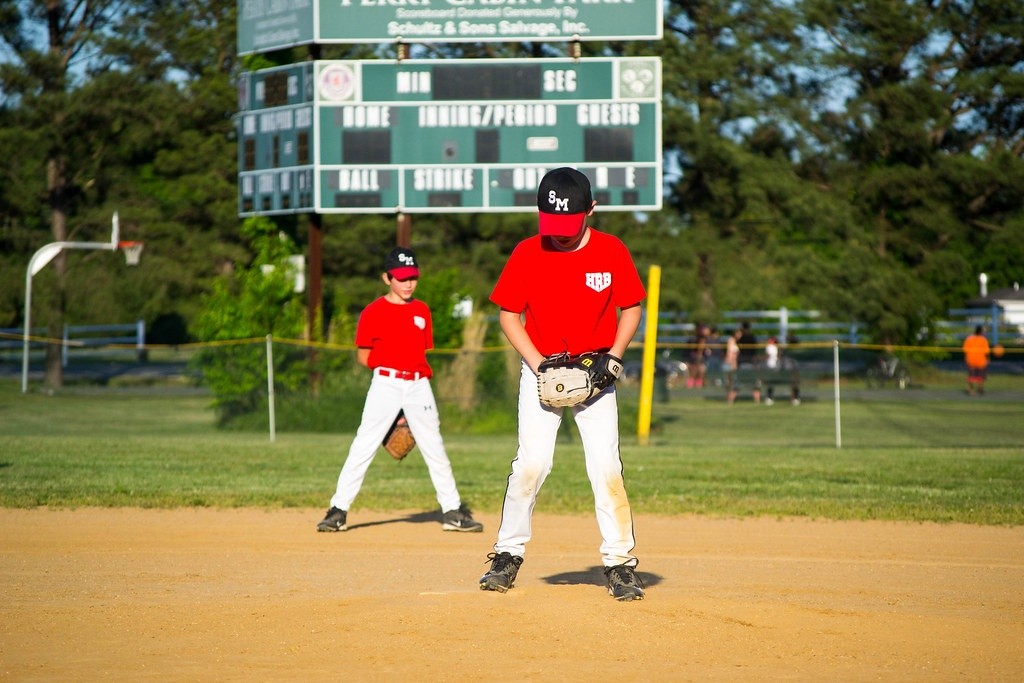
[966,387,975,395]
[978,389,985,395]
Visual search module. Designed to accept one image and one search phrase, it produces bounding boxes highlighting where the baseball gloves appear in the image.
[384,420,416,460]
[537,350,623,409]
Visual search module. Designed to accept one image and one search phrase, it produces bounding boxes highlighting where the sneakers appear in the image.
[603,558,645,600]
[442,510,482,532]
[479,553,524,594]
[317,506,348,531]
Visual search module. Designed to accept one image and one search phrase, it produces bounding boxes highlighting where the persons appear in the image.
[682,318,802,407]
[316,247,484,533]
[962,325,991,396]
[479,165,647,603]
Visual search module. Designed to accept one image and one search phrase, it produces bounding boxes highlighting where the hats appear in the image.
[537,167,592,237]
[385,246,420,280]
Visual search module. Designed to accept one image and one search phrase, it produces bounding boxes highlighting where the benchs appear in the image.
[726,369,800,406]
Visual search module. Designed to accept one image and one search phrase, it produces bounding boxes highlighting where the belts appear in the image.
[380,369,424,380]
